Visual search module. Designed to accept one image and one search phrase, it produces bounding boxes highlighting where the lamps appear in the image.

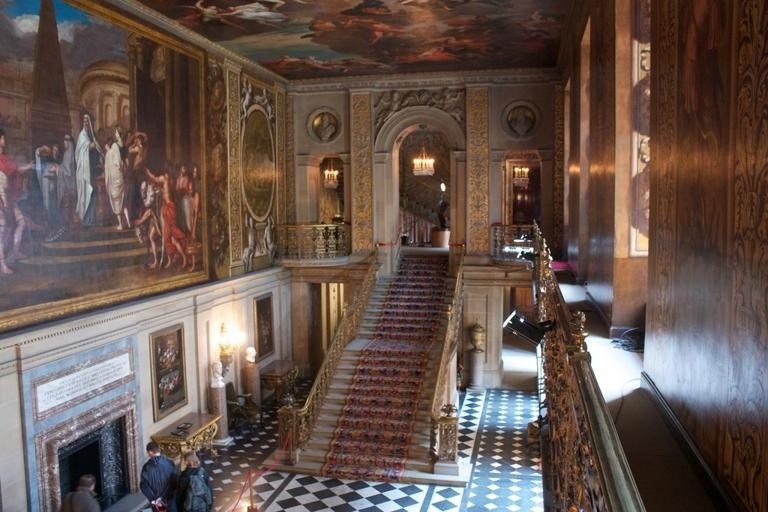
[513,167,530,188]
[501,309,557,347]
[217,318,246,378]
[323,157,338,188]
[412,123,435,176]
[517,250,539,261]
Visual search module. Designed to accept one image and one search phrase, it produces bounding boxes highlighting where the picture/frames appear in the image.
[0,0,212,336]
[253,291,275,363]
[148,323,188,423]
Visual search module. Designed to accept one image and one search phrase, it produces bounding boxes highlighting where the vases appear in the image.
[429,227,451,248]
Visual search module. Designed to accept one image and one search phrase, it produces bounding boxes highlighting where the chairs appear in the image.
[226,382,264,436]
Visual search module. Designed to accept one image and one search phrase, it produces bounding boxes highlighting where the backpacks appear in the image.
[182,468,213,512]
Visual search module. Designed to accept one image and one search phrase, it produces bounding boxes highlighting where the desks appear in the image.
[151,412,224,476]
[262,359,298,412]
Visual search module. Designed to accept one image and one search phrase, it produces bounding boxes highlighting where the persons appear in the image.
[58,443,213,512]
[0,114,200,275]
